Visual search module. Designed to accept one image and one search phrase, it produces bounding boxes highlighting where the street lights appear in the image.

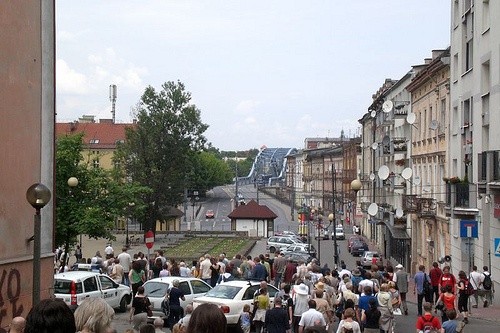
[317,206,333,266]
[124,202,135,248]
[307,204,315,254]
[332,163,362,270]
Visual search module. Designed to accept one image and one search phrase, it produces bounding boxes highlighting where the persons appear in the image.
[415,302,442,332]
[438,266,457,306]
[479,266,495,307]
[434,285,458,323]
[467,266,481,307]
[457,270,470,323]
[441,309,465,333]
[413,265,431,316]
[393,264,409,316]
[9,244,400,333]
[352,224,359,235]
[429,262,442,313]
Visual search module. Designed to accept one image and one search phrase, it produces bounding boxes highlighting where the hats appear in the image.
[395,264,403,269]
[352,270,361,276]
[292,283,310,295]
[314,282,325,291]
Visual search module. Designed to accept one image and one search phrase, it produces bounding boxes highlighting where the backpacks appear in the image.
[422,315,434,333]
[463,279,474,295]
[280,295,291,310]
[423,273,432,295]
[482,272,491,290]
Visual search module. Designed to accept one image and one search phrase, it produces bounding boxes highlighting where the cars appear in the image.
[323,227,345,240]
[193,281,288,324]
[136,276,214,323]
[265,231,318,273]
[348,235,384,271]
[206,210,214,219]
[53,263,132,314]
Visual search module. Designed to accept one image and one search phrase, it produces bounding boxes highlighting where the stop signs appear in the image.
[145,230,154,248]
